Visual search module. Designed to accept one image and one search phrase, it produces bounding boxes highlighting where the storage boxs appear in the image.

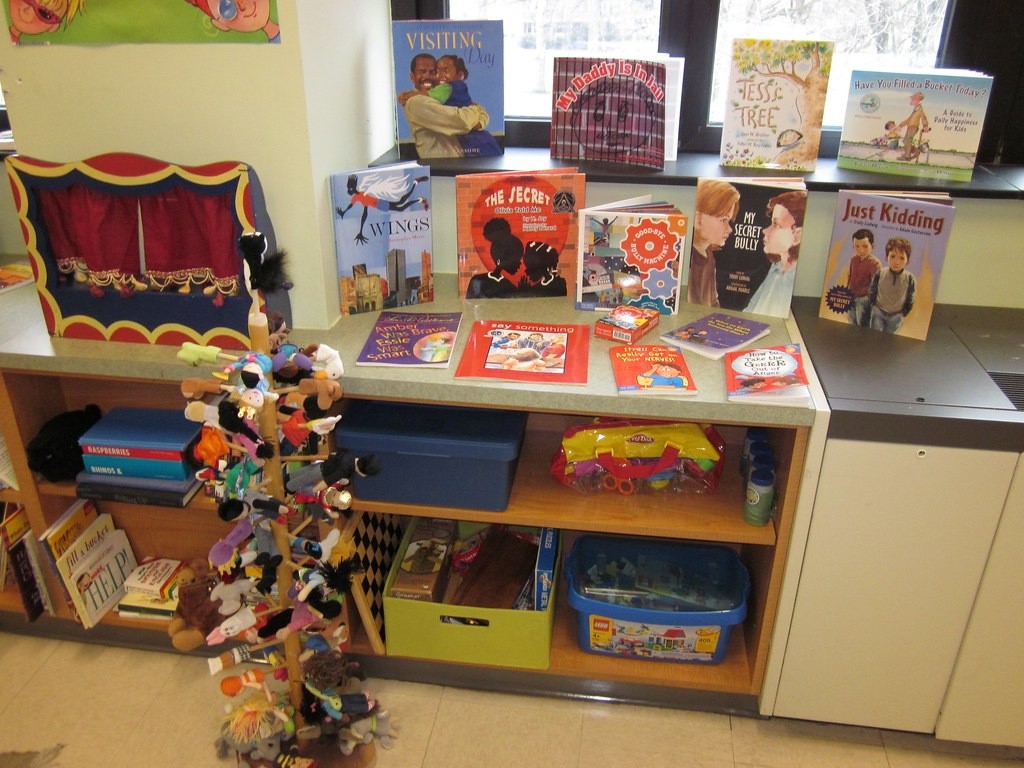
[333,399,532,515]
[559,530,752,668]
[382,515,564,673]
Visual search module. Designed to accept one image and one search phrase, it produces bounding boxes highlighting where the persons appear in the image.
[69,529,139,629]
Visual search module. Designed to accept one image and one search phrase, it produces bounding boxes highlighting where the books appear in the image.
[0,263,36,295]
[659,312,771,361]
[38,497,83,624]
[81,444,183,461]
[686,175,808,319]
[46,499,98,561]
[455,165,587,300]
[329,159,435,317]
[355,310,464,369]
[82,453,192,481]
[577,192,690,317]
[118,590,179,616]
[818,188,956,342]
[608,345,699,397]
[724,342,812,409]
[77,406,204,452]
[550,52,686,171]
[0,427,55,622]
[75,469,199,493]
[390,18,506,160]
[836,67,994,183]
[453,319,592,385]
[719,37,835,172]
[55,513,115,630]
[123,555,188,601]
[75,479,204,509]
[118,611,174,621]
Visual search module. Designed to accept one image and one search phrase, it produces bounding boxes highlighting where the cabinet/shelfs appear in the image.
[755,293,1023,751]
[0,270,834,722]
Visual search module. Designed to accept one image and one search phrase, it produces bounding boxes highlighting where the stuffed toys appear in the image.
[167,557,233,652]
[172,229,403,768]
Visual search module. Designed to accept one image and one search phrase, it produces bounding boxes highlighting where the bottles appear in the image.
[739,427,779,525]
